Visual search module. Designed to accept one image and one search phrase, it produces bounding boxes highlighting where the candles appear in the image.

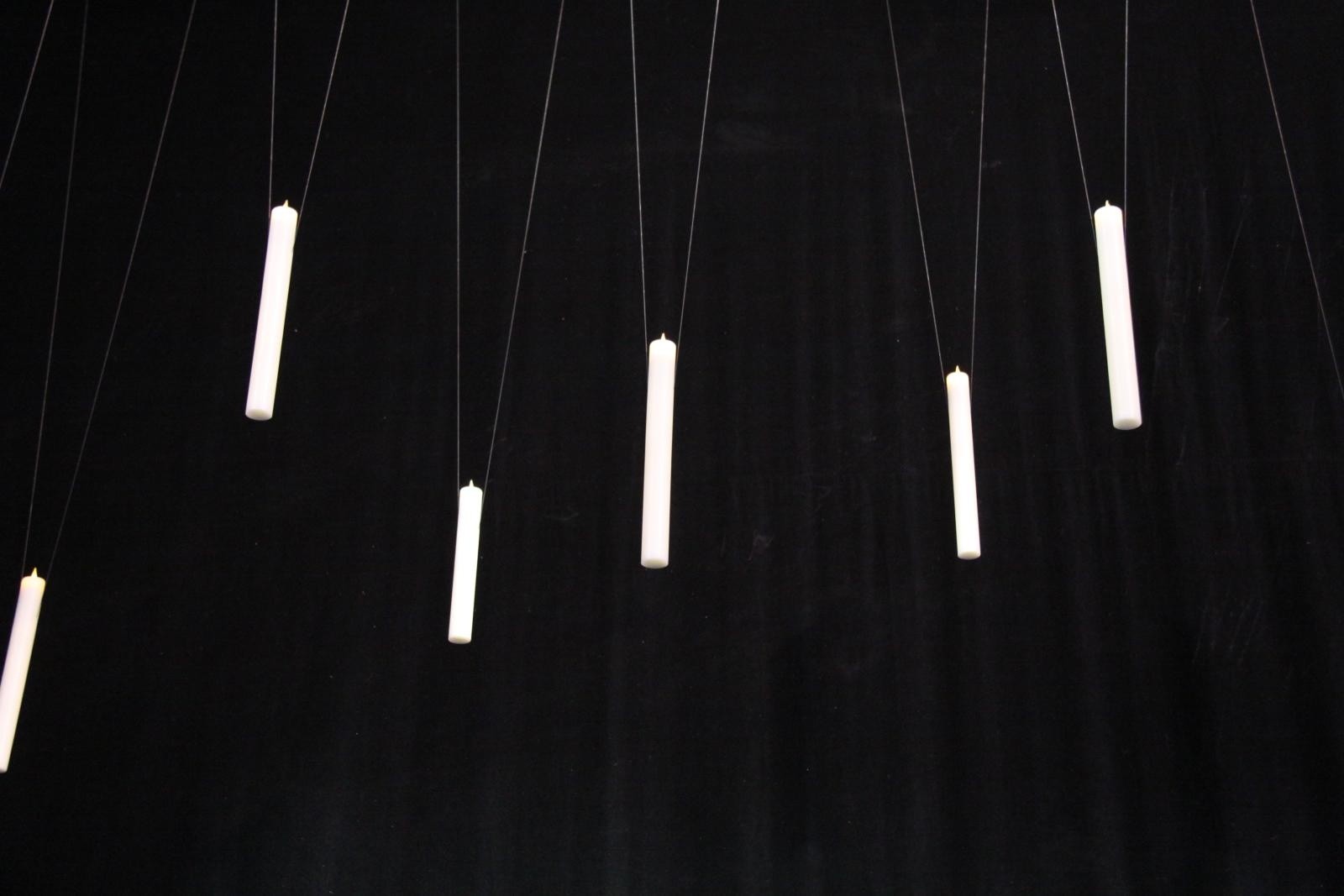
[946,366,981,560]
[641,330,676,569]
[448,477,482,643]
[0,568,46,774]
[1094,200,1142,430]
[246,200,298,421]
